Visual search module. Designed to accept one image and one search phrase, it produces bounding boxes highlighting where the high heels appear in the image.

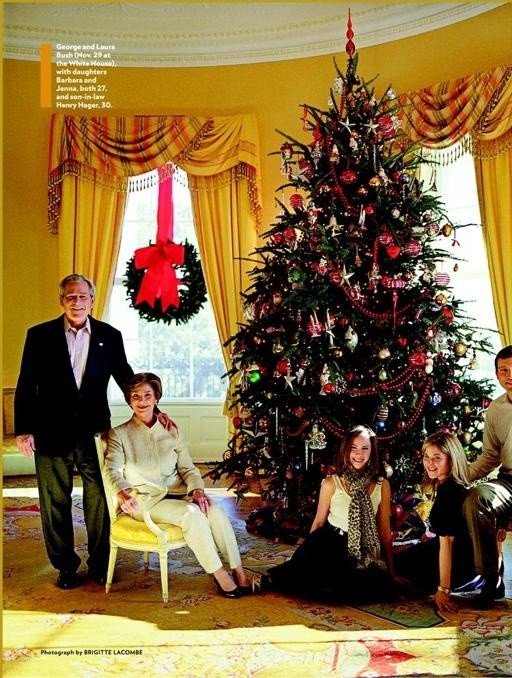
[214,571,251,598]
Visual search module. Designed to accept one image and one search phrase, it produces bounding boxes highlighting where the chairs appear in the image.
[94,431,192,604]
[1,387,38,476]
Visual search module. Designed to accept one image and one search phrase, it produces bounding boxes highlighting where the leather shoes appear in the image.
[451,589,491,609]
[57,573,116,589]
